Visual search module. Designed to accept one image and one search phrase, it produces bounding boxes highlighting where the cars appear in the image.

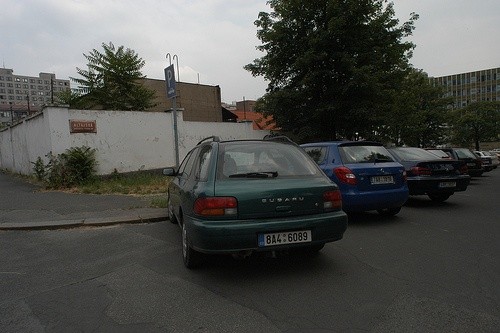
[387,147,470,203]
[435,148,500,179]
[167,136,348,269]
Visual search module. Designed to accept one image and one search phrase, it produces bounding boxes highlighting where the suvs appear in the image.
[299,141,410,217]
